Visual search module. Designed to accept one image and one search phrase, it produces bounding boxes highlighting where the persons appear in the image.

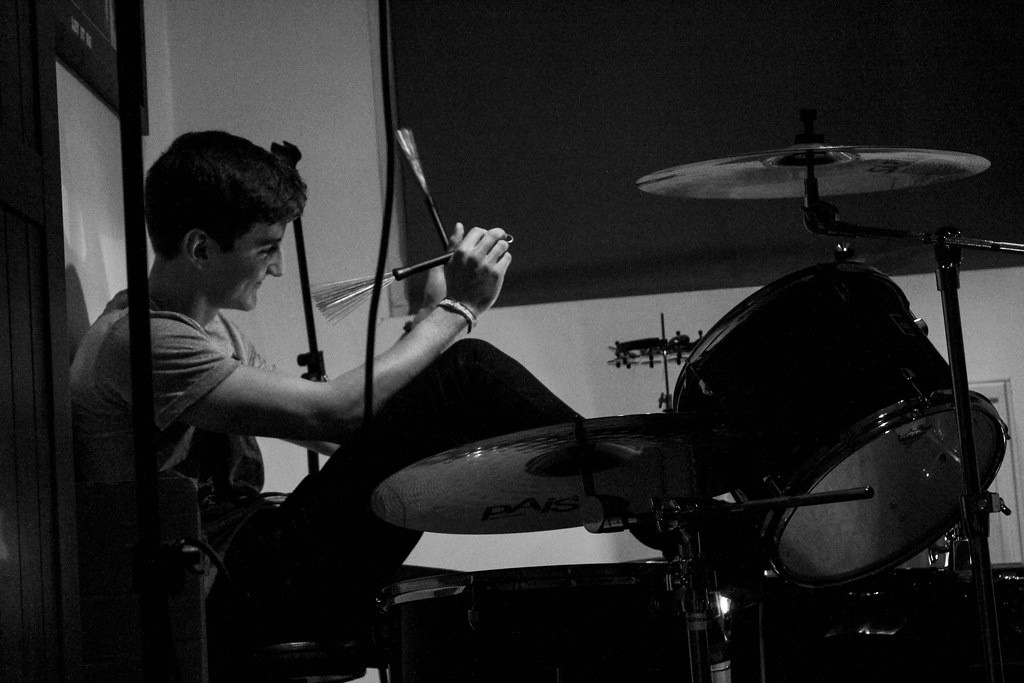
[69,130,757,646]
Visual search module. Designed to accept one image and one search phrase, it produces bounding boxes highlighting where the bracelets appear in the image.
[404,321,413,332]
[440,296,479,332]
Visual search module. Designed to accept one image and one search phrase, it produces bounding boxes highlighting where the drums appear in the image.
[375,562,692,683]
[673,261,1007,589]
[730,563,1024,683]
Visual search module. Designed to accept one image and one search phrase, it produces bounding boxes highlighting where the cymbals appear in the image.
[636,143,992,200]
[369,413,762,534]
